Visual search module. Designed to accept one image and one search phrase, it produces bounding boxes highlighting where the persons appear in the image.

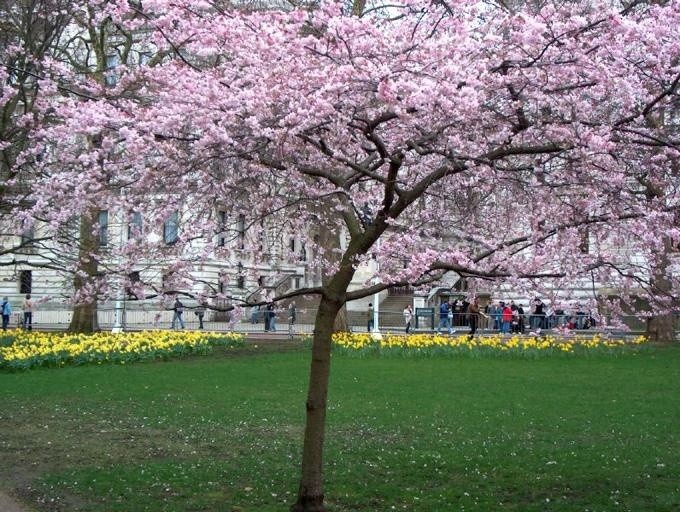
[21,295,33,331]
[435,297,594,339]
[367,303,373,331]
[403,304,416,334]
[269,303,279,333]
[264,304,271,332]
[195,307,205,329]
[0,295,12,332]
[288,301,297,324]
[248,299,260,325]
[170,298,185,329]
